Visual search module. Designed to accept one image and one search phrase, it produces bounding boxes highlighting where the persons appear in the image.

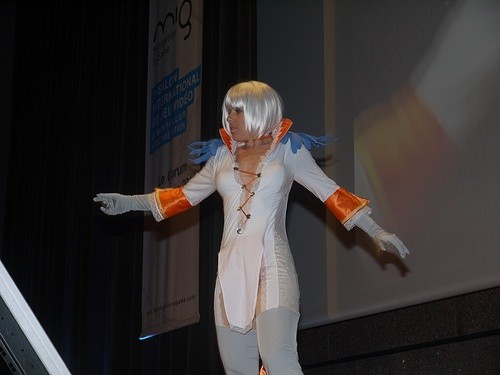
[93,81,410,374]
